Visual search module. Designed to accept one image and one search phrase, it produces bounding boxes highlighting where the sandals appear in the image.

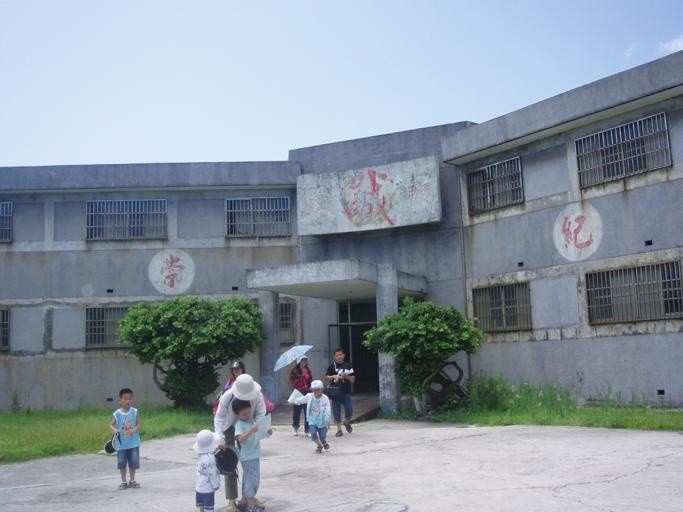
[316,424,351,454]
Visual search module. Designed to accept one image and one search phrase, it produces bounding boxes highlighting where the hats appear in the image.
[231,374,261,400]
[193,430,220,453]
[228,361,243,369]
[104,433,121,453]
[297,355,308,364]
[310,380,323,390]
[215,446,238,473]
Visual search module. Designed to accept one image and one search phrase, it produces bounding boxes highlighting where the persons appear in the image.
[289,354,313,434]
[214,372,266,511]
[230,399,274,510]
[324,346,358,438]
[194,430,222,512]
[223,363,245,385]
[308,381,333,451]
[110,387,142,489]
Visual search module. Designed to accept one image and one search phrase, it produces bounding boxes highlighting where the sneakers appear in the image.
[227,497,265,512]
[303,431,311,437]
[119,483,127,489]
[129,481,140,488]
[292,426,297,436]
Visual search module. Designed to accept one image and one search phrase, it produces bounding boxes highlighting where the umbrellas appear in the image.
[272,345,314,372]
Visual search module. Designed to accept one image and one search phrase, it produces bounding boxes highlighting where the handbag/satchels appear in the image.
[326,387,342,400]
